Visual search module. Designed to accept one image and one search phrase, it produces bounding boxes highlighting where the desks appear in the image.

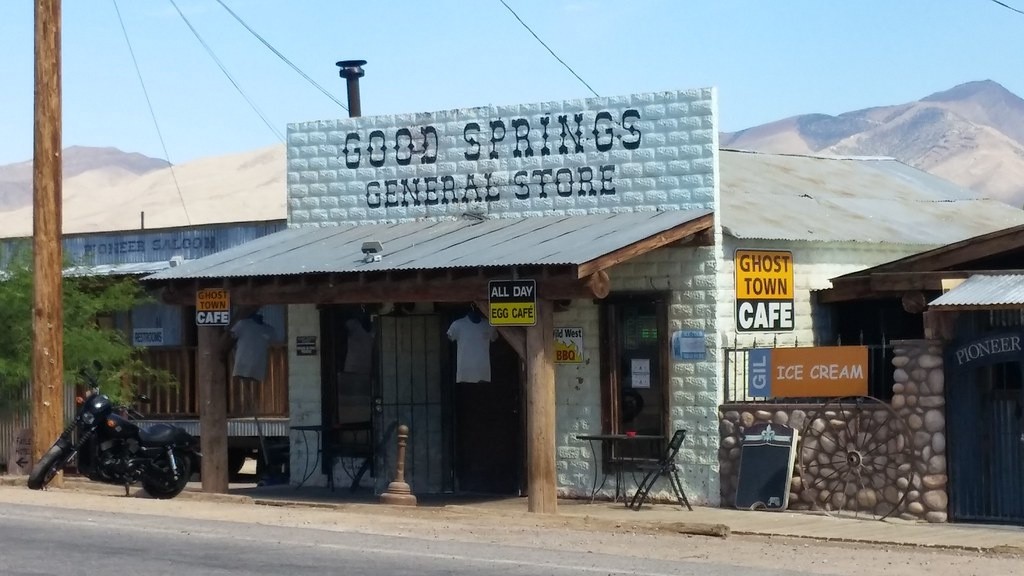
[291,424,371,493]
[576,435,665,507]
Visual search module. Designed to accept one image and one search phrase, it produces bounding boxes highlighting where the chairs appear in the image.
[631,429,692,511]
[332,421,398,492]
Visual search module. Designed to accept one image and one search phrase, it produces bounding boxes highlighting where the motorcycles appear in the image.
[26,358,204,501]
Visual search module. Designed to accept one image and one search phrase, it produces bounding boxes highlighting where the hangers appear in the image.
[356,304,375,323]
[467,303,485,323]
[252,305,267,324]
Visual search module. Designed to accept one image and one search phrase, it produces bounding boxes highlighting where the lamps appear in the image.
[361,241,383,263]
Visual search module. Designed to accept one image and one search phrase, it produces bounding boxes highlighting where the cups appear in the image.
[626,431,638,437]
